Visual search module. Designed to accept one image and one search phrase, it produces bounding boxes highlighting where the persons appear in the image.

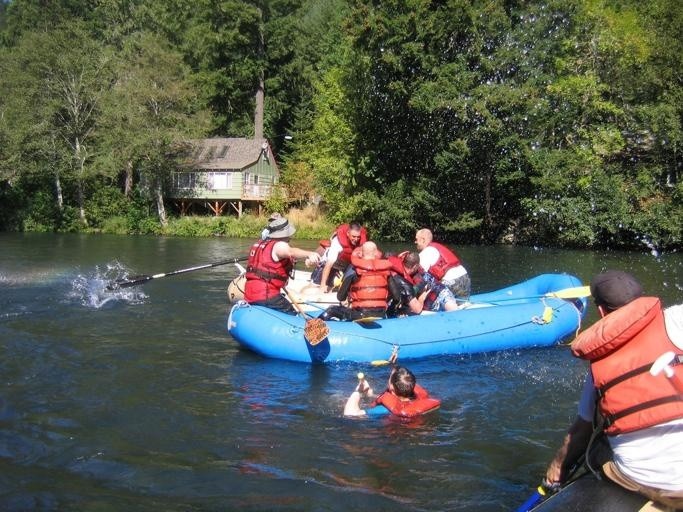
[546,269,683,512]
[309,221,368,293]
[389,251,431,317]
[243,217,320,314]
[260,212,281,241]
[342,366,428,417]
[413,229,470,299]
[396,252,469,311]
[315,242,399,321]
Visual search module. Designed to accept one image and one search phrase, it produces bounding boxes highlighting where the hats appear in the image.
[261,210,297,242]
[587,269,644,312]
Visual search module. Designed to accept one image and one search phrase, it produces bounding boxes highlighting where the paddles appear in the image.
[282,287,329,346]
[105,256,249,292]
[456,286,592,302]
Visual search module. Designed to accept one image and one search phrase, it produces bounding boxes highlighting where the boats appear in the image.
[228,345,573,431]
[219,267,589,367]
[511,461,683,512]
